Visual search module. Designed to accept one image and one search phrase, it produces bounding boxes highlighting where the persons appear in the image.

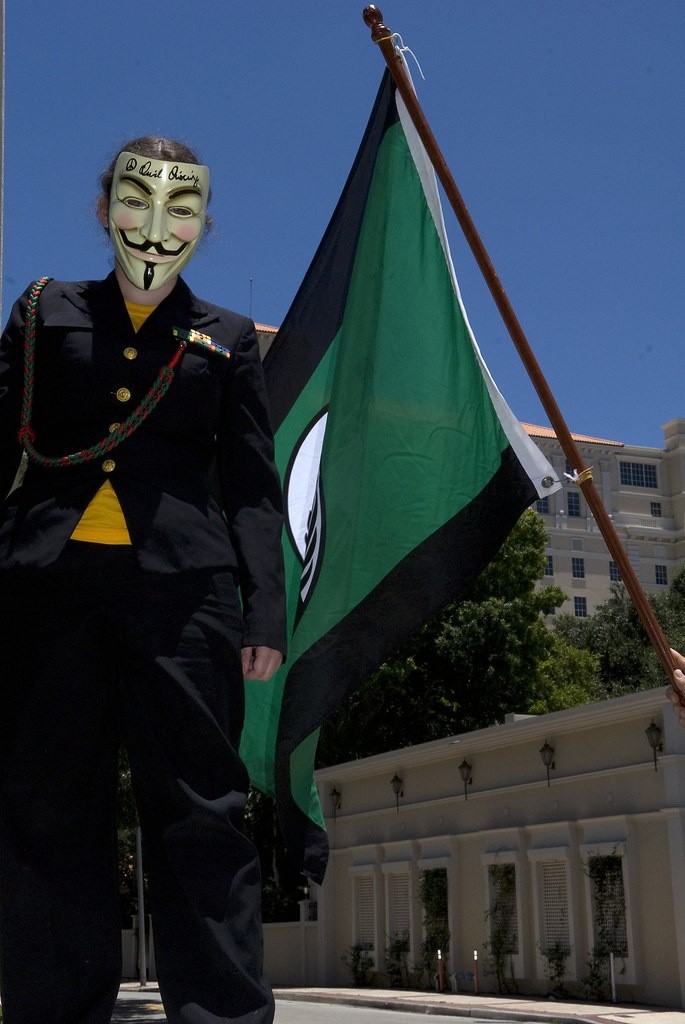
[663,647,685,728]
[0,136,291,1024]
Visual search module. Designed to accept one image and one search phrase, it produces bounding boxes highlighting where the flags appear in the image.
[238,59,568,905]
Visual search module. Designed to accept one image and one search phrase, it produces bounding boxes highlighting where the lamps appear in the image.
[644,718,663,771]
[458,758,472,801]
[329,786,340,823]
[538,739,555,787]
[391,770,403,811]
